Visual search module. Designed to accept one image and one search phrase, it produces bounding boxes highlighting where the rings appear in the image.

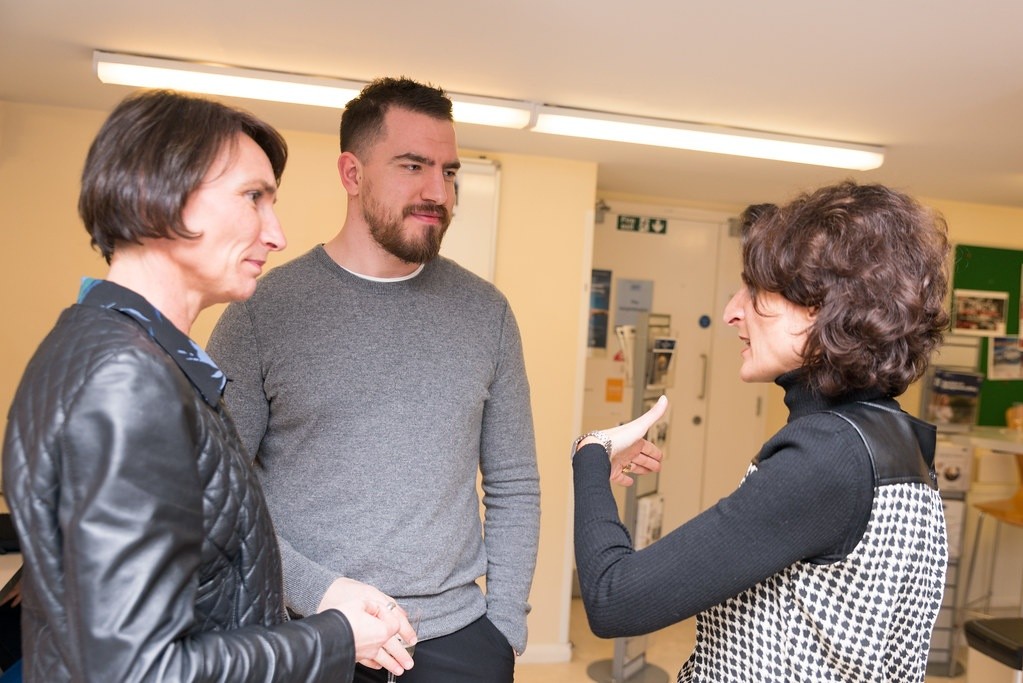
[387,601,397,610]
[622,462,632,474]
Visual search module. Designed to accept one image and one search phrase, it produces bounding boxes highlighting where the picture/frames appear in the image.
[951,287,1011,339]
[986,334,1023,381]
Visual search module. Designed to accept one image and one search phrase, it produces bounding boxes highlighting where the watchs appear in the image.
[571,428,613,462]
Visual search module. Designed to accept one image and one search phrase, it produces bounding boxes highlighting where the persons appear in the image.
[206,74,540,683]
[572,180,954,682]
[4,88,416,682]
